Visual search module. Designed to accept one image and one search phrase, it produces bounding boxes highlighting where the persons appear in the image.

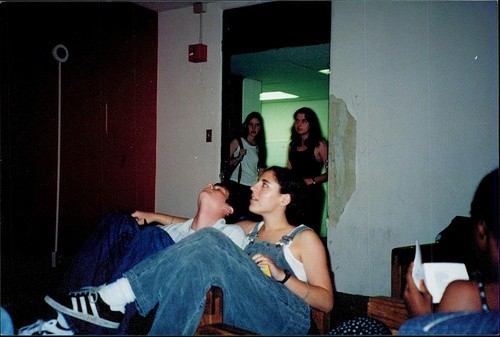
[227,112,268,202]
[20,182,248,337]
[45,167,335,337]
[332,168,500,337]
[287,106,328,234]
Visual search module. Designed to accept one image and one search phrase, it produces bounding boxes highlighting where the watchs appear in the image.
[277,269,292,284]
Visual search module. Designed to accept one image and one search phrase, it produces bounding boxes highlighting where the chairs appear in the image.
[194,239,331,336]
[366,243,440,336]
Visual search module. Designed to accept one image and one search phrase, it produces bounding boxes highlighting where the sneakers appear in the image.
[44,283,126,328]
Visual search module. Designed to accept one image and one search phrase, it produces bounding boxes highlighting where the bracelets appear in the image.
[171,216,175,224]
[312,178,316,185]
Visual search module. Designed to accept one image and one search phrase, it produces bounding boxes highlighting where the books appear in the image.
[412,241,469,303]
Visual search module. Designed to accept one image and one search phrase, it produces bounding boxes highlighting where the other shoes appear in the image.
[18,319,76,335]
